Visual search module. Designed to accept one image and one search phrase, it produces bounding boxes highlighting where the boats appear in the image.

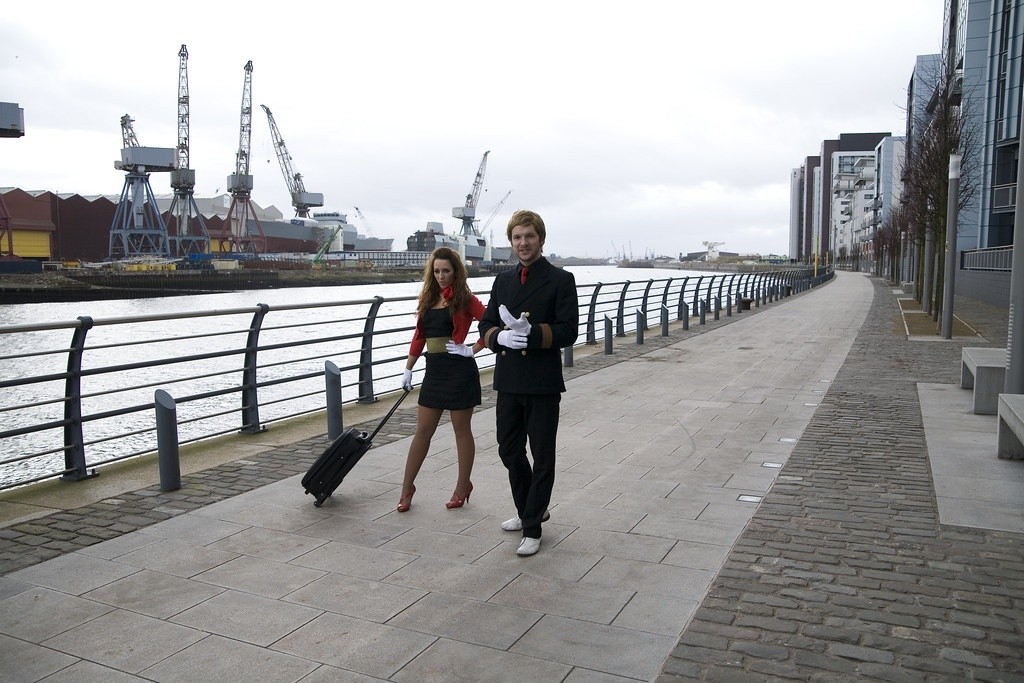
[612,240,654,269]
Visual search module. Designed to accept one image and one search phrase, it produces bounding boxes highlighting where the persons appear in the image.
[397,248,488,512]
[478,211,579,555]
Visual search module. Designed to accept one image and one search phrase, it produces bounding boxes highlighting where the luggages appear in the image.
[300,385,414,508]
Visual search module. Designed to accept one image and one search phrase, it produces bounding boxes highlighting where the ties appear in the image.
[520,268,528,285]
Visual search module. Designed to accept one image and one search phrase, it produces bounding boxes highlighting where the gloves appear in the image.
[445,340,475,357]
[401,367,412,392]
[496,329,528,350]
[498,305,532,335]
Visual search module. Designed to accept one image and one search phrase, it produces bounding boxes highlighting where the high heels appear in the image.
[397,485,416,512]
[446,481,473,509]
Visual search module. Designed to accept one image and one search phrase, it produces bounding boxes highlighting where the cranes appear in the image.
[450,149,514,240]
[104,41,324,257]
[351,203,374,239]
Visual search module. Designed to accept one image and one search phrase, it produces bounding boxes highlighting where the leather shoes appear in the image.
[501,503,551,531]
[516,533,543,557]
